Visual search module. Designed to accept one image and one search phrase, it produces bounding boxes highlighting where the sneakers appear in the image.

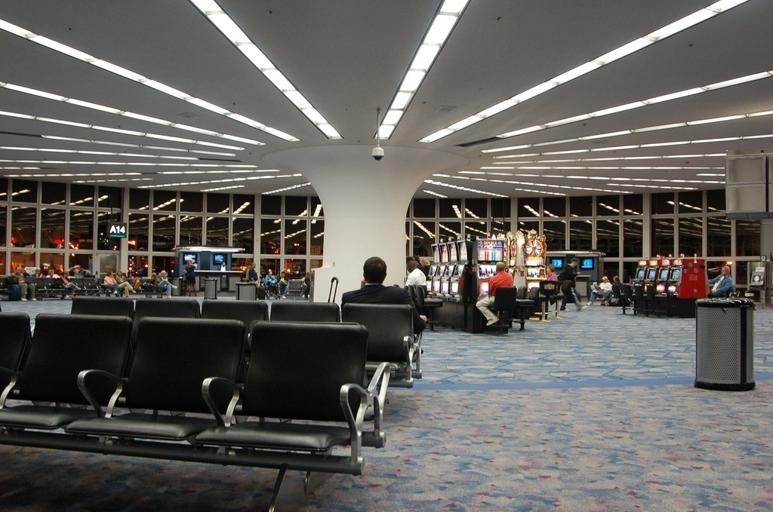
[282,296,286,299]
[577,306,587,311]
[486,317,500,326]
[587,302,593,306]
[21,297,27,301]
[561,309,569,312]
[30,298,37,301]
[167,296,172,299]
[172,285,177,289]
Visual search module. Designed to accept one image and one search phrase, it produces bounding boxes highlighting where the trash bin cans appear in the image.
[694,297,757,392]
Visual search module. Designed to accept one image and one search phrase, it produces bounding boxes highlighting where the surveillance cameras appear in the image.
[371,146,386,163]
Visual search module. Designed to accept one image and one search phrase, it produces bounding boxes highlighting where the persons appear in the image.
[249,263,258,281]
[586,276,612,306]
[264,269,278,297]
[179,260,196,296]
[476,262,514,326]
[31,268,45,278]
[59,272,81,290]
[406,259,427,286]
[45,269,60,278]
[341,257,428,334]
[708,266,732,298]
[121,273,127,280]
[278,271,288,299]
[529,264,559,301]
[12,266,37,302]
[104,272,120,294]
[612,275,626,306]
[240,264,252,282]
[155,271,177,298]
[115,271,136,298]
[558,258,587,311]
[219,262,227,271]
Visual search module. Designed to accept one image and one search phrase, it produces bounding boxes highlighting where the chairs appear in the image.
[634,286,653,316]
[416,286,442,332]
[647,285,668,318]
[409,285,426,315]
[516,287,527,300]
[623,284,641,316]
[515,287,540,331]
[488,286,516,336]
[612,286,628,315]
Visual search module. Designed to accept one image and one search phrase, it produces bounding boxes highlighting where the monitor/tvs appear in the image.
[647,269,656,282]
[548,257,564,269]
[477,240,503,260]
[432,247,439,263]
[446,245,455,262]
[458,242,466,261]
[213,253,228,267]
[579,258,593,270]
[184,250,198,266]
[671,269,682,281]
[659,269,668,280]
[439,246,447,263]
[636,268,645,283]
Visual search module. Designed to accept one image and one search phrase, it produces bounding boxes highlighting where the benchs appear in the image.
[306,361,390,491]
[366,330,423,378]
[286,279,308,297]
[0,277,99,299]
[98,279,166,298]
[71,297,414,417]
[0,312,370,512]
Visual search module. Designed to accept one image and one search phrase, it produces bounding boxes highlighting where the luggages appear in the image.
[7,284,21,301]
